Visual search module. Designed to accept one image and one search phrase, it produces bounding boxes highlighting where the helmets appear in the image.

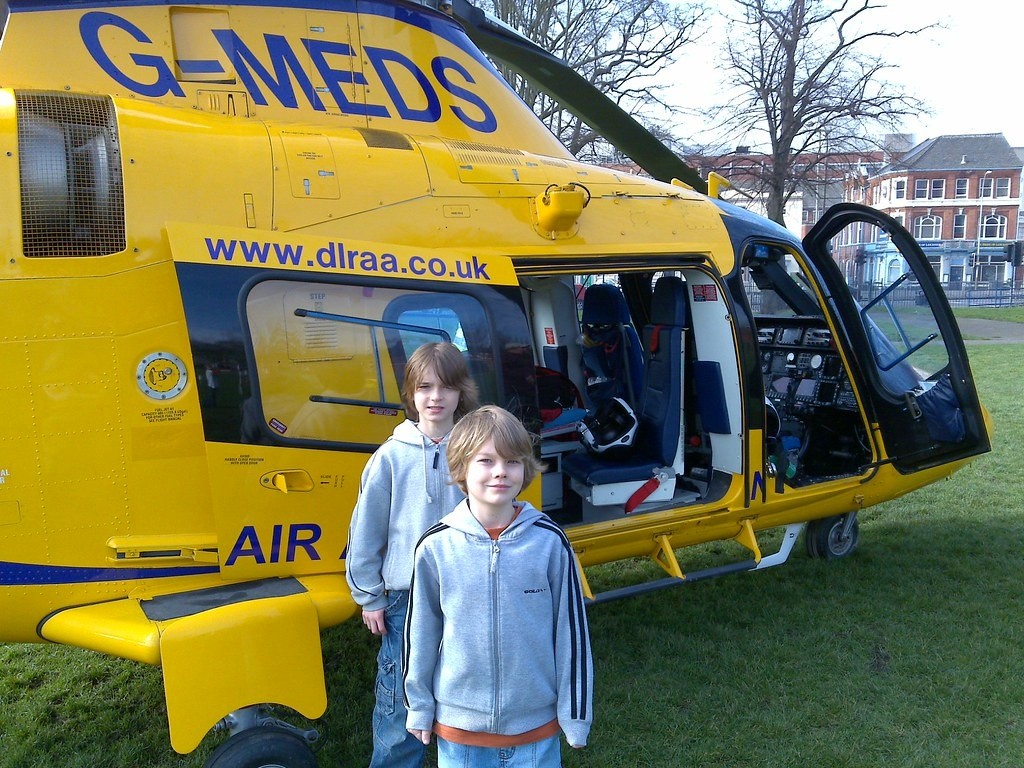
[577,396,644,463]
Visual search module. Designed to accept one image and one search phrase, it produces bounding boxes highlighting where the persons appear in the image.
[347,340,478,768]
[402,404,597,768]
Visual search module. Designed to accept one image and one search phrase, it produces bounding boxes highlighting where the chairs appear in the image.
[562,276,687,505]
[540,408,586,437]
[581,277,645,411]
[684,281,810,458]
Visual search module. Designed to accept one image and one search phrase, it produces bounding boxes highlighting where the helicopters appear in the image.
[2,1,996,767]
